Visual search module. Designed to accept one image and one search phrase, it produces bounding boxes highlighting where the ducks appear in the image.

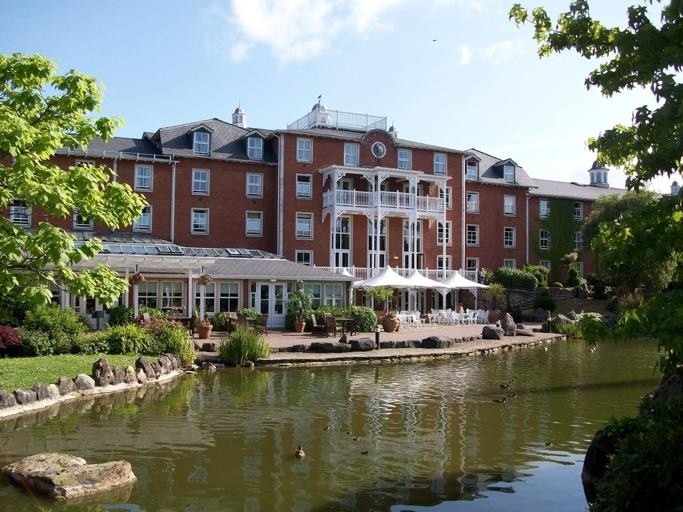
[295,444,305,457]
[324,425,329,430]
[347,430,359,441]
[488,375,519,404]
[361,451,368,454]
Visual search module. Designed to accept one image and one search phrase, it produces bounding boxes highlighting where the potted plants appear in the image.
[286,280,313,332]
[195,311,213,339]
[486,283,504,322]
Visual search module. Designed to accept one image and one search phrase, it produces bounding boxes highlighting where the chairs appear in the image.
[397,307,489,328]
[227,312,269,336]
[311,312,360,338]
